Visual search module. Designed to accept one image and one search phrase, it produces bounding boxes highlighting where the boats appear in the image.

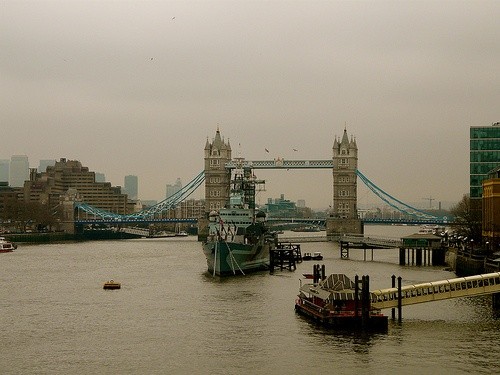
[312,252,323,260]
[0,237,18,254]
[294,264,383,328]
[303,252,312,261]
[104,279,120,290]
[302,272,327,279]
[200,163,281,278]
